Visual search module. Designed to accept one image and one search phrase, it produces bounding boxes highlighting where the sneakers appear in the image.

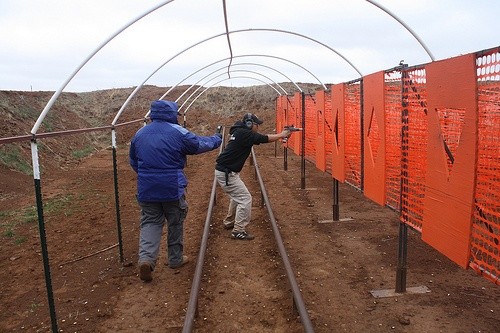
[232,231,254,239]
[224,224,234,229]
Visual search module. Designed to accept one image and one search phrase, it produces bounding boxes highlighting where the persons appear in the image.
[215,113,291,240]
[128,100,223,281]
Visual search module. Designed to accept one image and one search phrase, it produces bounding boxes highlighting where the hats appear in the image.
[244,112,263,124]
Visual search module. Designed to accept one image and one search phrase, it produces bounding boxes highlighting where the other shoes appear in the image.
[182,255,189,263]
[140,263,153,281]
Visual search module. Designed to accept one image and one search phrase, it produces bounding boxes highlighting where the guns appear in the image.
[282,125,303,143]
[216,126,223,133]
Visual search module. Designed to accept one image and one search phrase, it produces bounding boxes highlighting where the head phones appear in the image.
[245,113,253,129]
